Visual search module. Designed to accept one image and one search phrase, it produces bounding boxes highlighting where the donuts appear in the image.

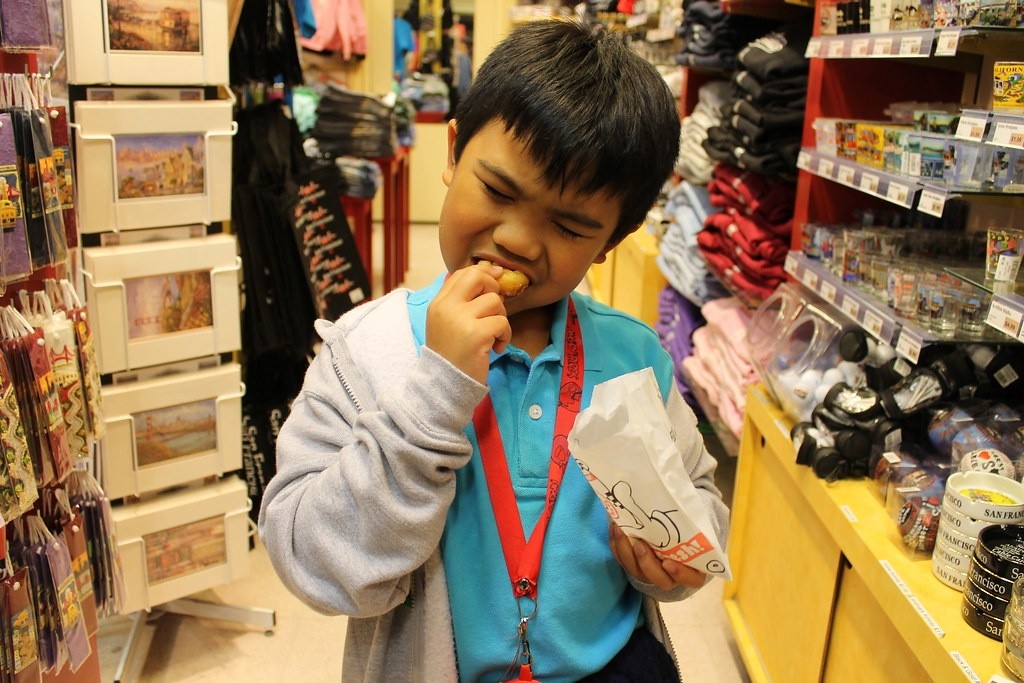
[479,259,530,297]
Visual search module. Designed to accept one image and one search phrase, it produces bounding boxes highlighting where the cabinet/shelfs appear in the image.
[719,27,1024,683]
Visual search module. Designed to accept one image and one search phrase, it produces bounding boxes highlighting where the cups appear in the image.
[974,145,995,180]
[994,147,1024,189]
[943,140,979,185]
[802,209,1024,340]
[961,525,1024,643]
[1000,579,1024,683]
[931,472,1024,592]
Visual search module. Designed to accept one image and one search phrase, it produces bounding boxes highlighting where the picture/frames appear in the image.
[62,0,229,86]
[100,361,239,500]
[73,99,232,234]
[83,232,243,375]
[113,475,249,617]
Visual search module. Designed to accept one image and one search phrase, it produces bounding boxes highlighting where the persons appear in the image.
[258,20,728,683]
